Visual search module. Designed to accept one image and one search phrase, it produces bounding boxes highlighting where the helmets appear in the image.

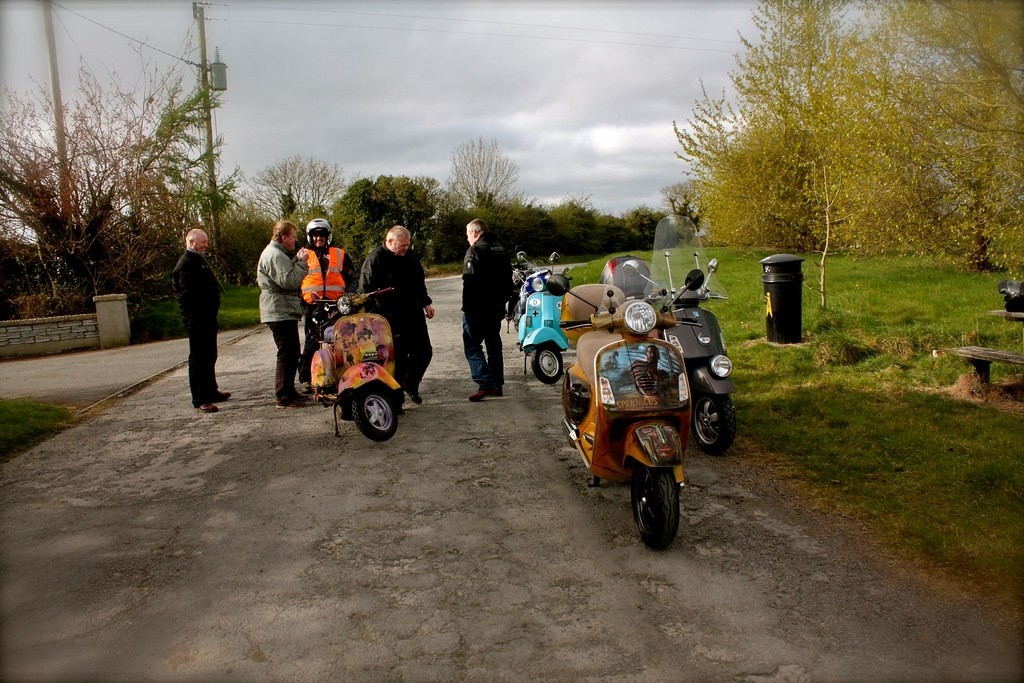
[998,280,1024,312]
[307,218,333,246]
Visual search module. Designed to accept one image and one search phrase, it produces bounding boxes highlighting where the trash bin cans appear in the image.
[760,254,804,344]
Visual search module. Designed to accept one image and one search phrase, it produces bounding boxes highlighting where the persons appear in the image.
[172,229,232,412]
[461,219,513,402]
[257,221,309,408]
[359,225,435,415]
[298,218,356,393]
[616,345,677,395]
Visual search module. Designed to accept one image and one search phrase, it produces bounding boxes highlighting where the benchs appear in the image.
[950,345,1024,385]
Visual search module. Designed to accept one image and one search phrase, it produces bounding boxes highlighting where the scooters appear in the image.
[544,268,705,551]
[514,268,569,332]
[517,252,572,385]
[309,286,405,443]
[598,215,738,456]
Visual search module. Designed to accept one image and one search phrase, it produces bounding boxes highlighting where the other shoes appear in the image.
[404,381,422,404]
[299,381,314,394]
[469,386,503,402]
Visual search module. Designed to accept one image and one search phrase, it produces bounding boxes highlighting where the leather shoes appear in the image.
[276,395,310,409]
[196,404,217,412]
[212,392,231,402]
